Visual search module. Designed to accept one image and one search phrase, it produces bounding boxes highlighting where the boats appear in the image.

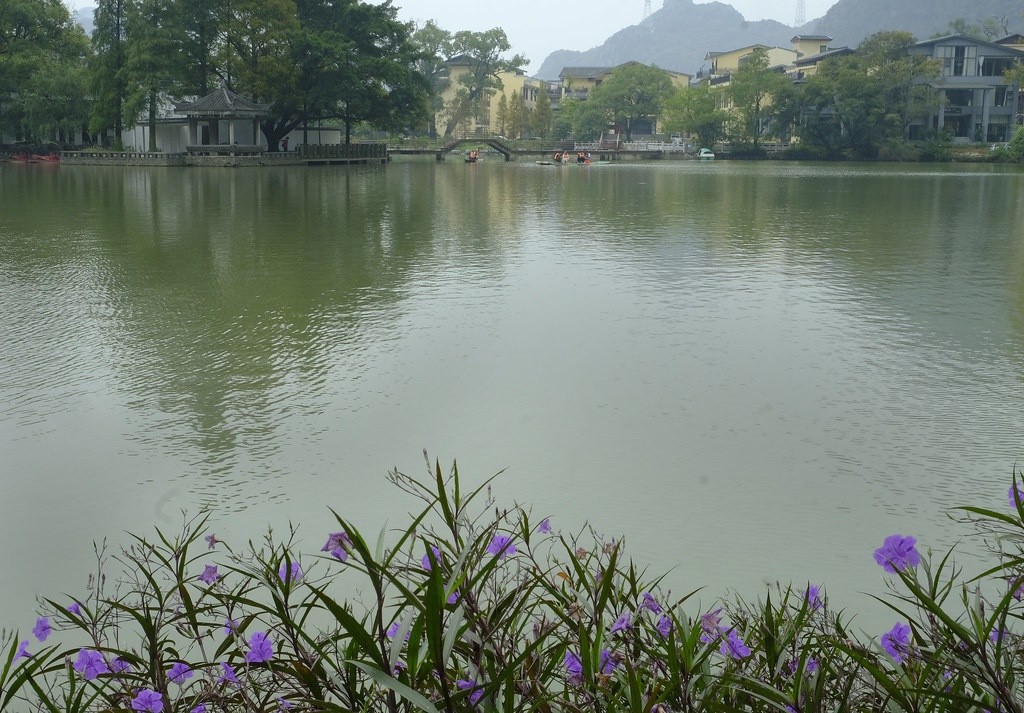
[536,161,611,165]
[465,159,477,162]
[697,148,715,157]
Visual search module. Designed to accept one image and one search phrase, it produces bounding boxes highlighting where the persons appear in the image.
[470,149,479,158]
[554,151,570,162]
[281,137,290,151]
